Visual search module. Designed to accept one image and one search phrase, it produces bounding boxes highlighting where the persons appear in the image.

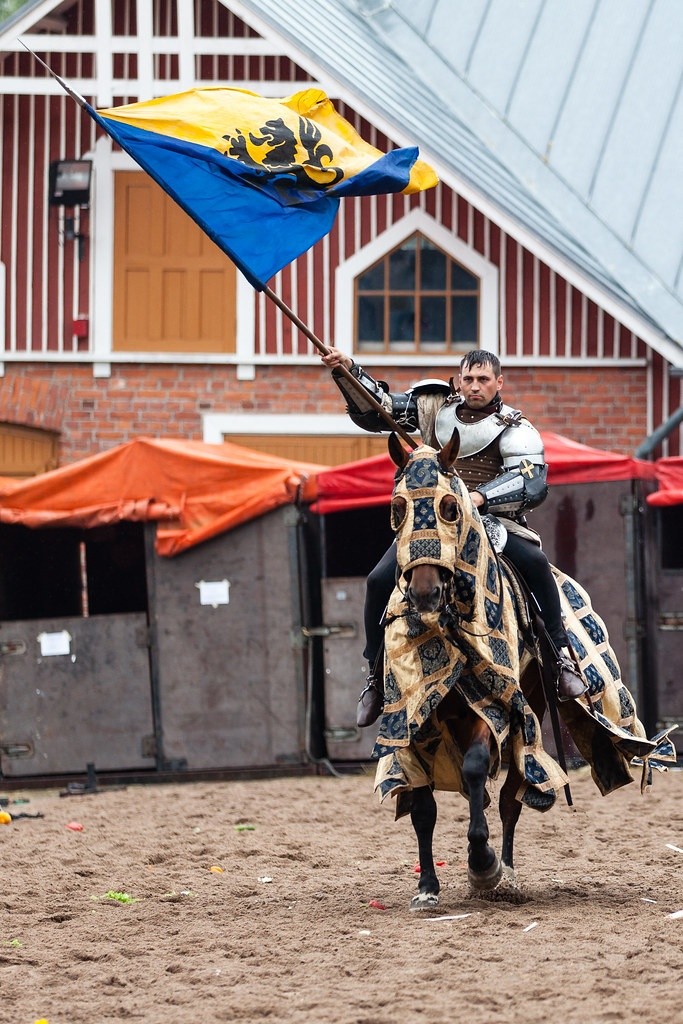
[321,342,590,728]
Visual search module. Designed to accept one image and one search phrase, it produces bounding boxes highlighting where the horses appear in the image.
[369,427,551,914]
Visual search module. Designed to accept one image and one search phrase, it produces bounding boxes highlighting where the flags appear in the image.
[90,83,441,293]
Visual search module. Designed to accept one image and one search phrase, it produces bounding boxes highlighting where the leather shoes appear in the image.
[355,670,388,728]
[549,659,584,698]
